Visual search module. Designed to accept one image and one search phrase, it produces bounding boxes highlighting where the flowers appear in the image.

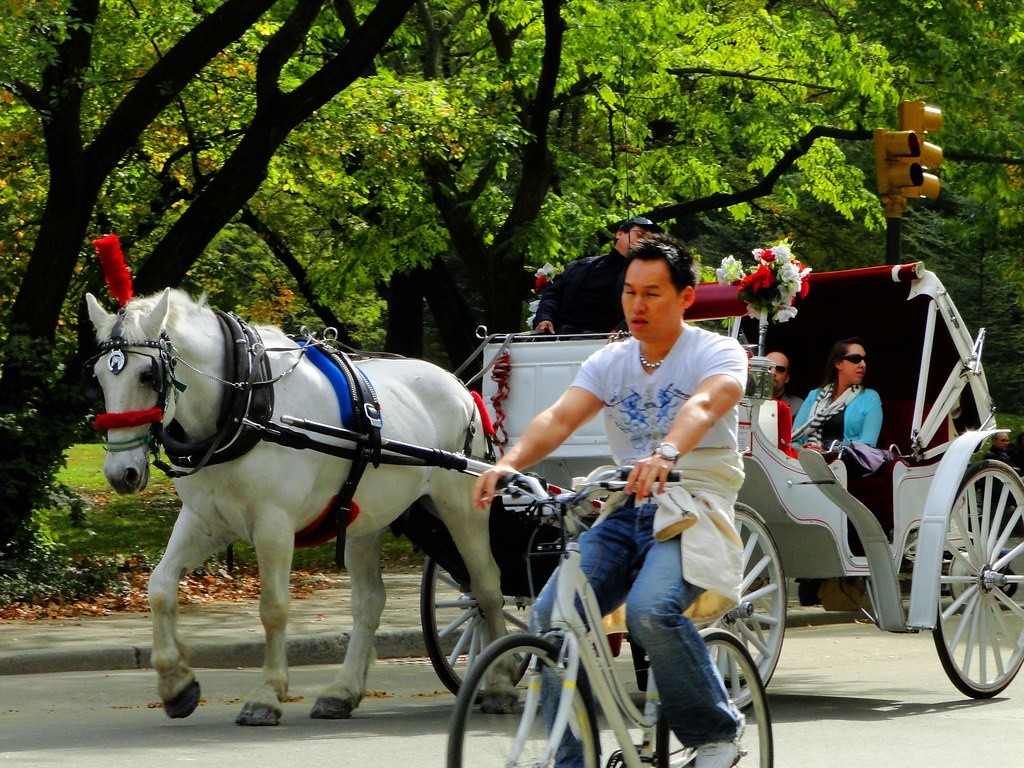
[525,261,563,327]
[700,236,814,329]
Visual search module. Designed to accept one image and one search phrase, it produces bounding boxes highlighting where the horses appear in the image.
[84,287,521,725]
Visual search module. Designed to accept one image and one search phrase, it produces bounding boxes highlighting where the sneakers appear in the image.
[694,699,746,768]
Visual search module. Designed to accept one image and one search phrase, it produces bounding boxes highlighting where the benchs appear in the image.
[858,402,949,538]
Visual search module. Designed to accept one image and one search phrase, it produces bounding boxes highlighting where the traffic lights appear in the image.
[874,124,926,195]
[899,97,943,203]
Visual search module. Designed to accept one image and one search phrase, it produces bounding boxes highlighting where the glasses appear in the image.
[769,365,786,373]
[839,354,868,364]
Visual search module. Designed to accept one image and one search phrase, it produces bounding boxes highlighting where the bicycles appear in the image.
[445,466,779,768]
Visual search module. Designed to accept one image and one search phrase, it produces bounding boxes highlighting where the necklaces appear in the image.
[640,352,663,367]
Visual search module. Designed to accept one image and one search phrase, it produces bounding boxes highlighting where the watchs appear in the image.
[653,443,681,464]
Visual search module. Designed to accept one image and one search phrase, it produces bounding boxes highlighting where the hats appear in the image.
[619,217,665,235]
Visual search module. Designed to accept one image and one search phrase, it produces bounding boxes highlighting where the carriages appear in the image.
[76,236,1024,731]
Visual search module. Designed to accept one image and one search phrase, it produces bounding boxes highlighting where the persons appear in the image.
[767,350,805,419]
[471,234,750,768]
[979,432,1022,535]
[531,216,667,338]
[792,336,882,460]
